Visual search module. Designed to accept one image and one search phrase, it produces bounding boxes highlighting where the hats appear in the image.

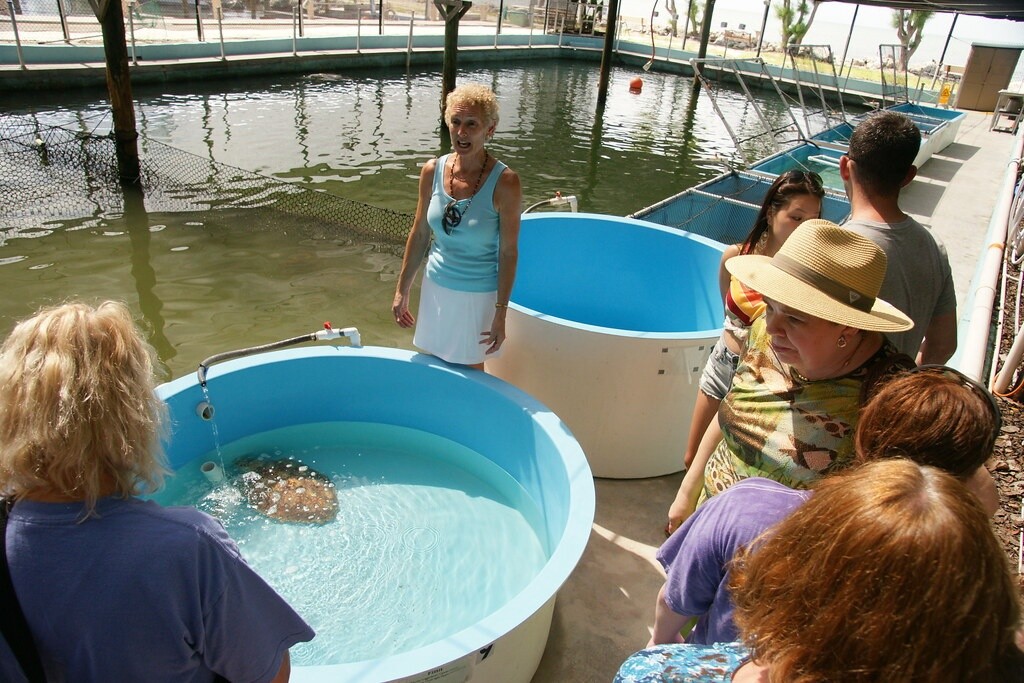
[725,219,915,333]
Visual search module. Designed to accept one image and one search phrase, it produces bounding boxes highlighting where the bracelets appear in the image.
[496,304,507,308]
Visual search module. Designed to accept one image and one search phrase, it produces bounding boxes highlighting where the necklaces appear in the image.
[449,151,488,219]
[795,338,865,383]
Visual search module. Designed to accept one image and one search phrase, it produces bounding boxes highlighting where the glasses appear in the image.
[902,362,1002,438]
[772,169,824,192]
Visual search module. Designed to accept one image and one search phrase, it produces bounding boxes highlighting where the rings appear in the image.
[401,316,404,320]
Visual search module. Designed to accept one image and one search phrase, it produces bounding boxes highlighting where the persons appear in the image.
[610,454,1024,683]
[0,298,315,683]
[836,109,957,368]
[684,169,825,462]
[663,220,1000,537]
[645,364,1004,647]
[390,84,523,372]
